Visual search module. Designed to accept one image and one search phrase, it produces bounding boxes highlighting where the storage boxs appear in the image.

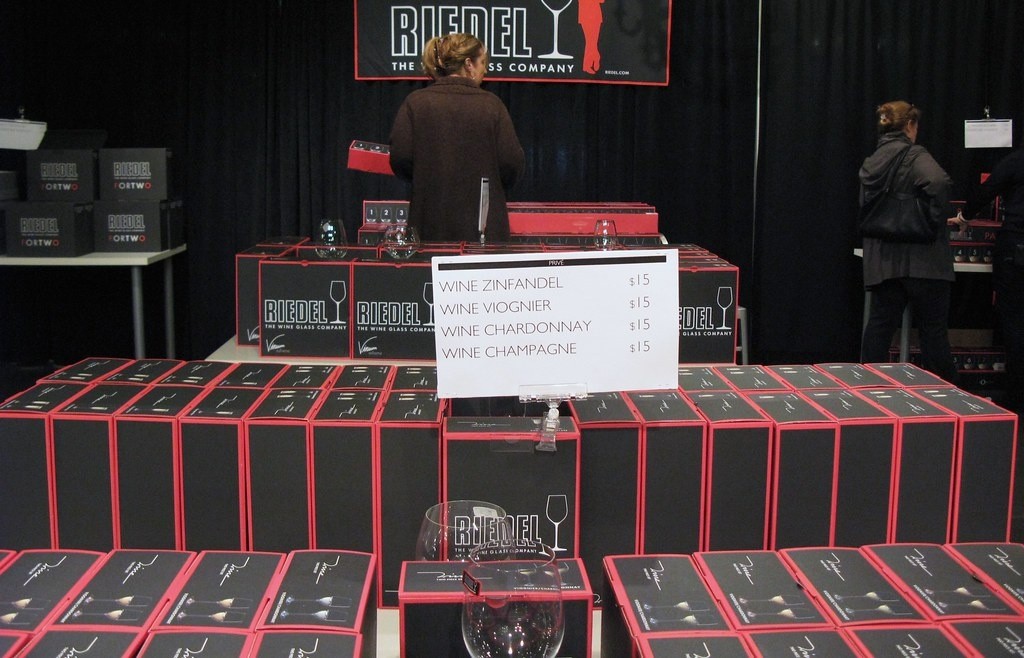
[0,146,1024,658]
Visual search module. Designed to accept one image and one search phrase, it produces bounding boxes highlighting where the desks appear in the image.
[0,243,187,358]
[206,335,435,367]
[853,249,993,362]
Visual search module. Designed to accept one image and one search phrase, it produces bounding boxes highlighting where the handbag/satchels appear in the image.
[856,145,947,244]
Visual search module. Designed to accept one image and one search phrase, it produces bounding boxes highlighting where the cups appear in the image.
[313,219,349,258]
[461,540,565,657]
[383,225,420,259]
[415,499,516,561]
[594,219,618,249]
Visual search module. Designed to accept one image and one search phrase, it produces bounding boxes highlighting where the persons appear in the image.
[388,32,527,243]
[947,153,1024,412]
[857,101,958,386]
